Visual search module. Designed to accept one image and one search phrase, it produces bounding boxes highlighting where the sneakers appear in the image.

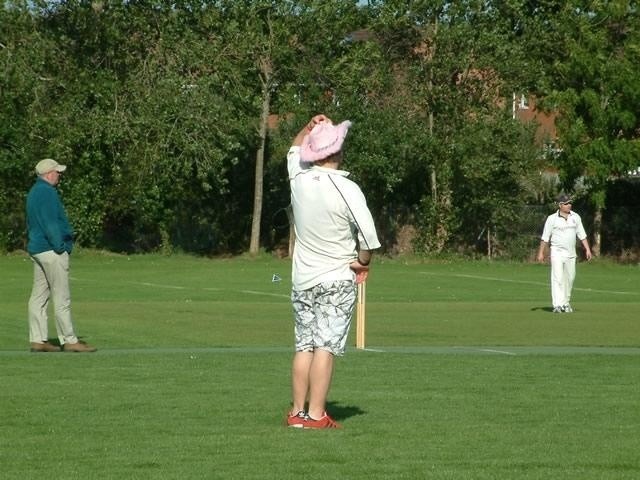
[304,408,344,429]
[31,341,61,352]
[287,410,305,428]
[64,341,97,352]
[552,304,573,313]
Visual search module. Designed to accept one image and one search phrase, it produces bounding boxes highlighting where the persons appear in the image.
[535,194,593,313]
[286,112,382,431]
[25,158,96,352]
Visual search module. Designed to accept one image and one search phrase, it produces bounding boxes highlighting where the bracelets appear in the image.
[357,256,371,266]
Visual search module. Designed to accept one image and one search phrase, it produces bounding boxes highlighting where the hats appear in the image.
[35,159,67,174]
[558,195,573,204]
[300,120,352,163]
